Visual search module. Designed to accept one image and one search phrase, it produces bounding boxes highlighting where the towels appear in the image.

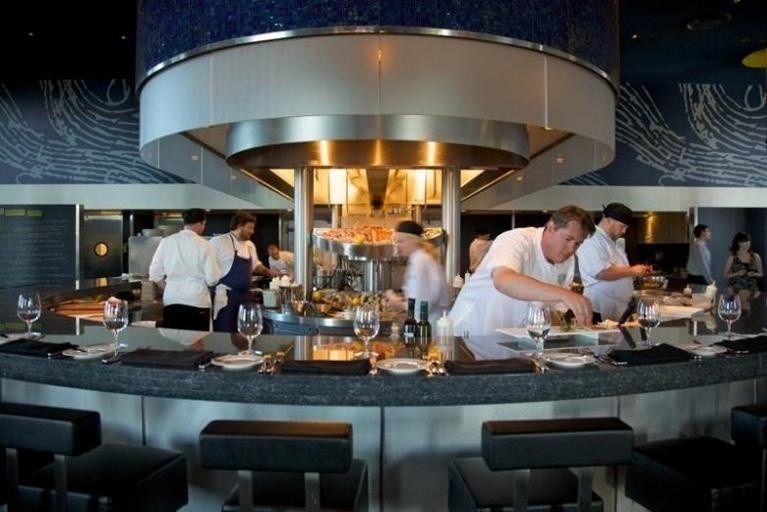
[608,342,690,366]
[281,358,371,378]
[0,337,79,358]
[119,348,213,371]
[715,335,767,354]
[444,358,535,375]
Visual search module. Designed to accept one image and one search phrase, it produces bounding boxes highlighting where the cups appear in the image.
[637,297,661,349]
[16,293,41,340]
[237,304,263,355]
[526,300,551,354]
[718,294,742,338]
[351,306,380,359]
[103,300,129,360]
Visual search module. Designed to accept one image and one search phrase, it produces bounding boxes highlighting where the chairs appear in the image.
[448,416,634,512]
[0,403,188,512]
[627,403,767,512]
[199,419,369,511]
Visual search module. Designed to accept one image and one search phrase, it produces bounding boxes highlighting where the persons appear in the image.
[447,205,593,335]
[269,244,295,273]
[387,220,449,335]
[576,202,652,326]
[686,224,714,285]
[724,231,763,321]
[210,213,288,334]
[148,208,220,332]
[469,225,493,273]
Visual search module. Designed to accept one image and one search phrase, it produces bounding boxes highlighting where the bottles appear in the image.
[436,310,453,363]
[682,284,693,306]
[416,300,431,350]
[705,281,717,299]
[403,298,416,348]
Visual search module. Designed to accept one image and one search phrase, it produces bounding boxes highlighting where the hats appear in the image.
[394,220,424,237]
[602,202,641,227]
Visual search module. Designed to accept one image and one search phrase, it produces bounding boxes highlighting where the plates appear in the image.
[542,352,595,368]
[686,345,727,356]
[376,358,428,376]
[62,346,112,359]
[210,355,263,371]
